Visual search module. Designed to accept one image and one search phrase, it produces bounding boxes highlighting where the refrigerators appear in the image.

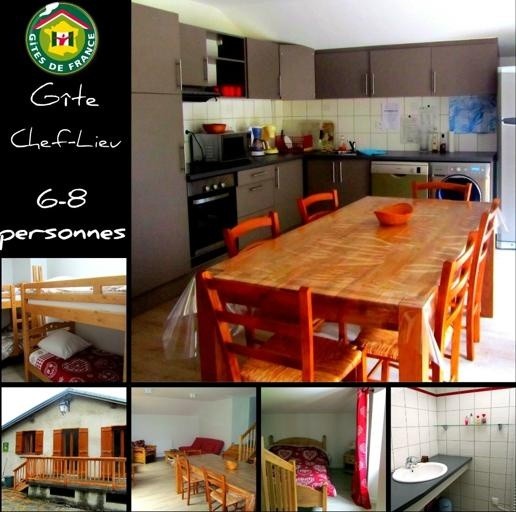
[495,65,516,250]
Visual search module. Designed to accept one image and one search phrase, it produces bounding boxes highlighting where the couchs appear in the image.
[179,438,224,456]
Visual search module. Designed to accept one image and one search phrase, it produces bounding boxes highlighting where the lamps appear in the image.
[58,400,69,414]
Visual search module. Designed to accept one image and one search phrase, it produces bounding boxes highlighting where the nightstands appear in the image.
[342,449,355,472]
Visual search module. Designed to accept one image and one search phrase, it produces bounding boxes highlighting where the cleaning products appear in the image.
[475,416,480,426]
[373,202,414,226]
[464,415,469,425]
[481,414,488,424]
[468,413,474,425]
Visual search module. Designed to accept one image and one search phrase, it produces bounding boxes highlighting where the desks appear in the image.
[181,453,256,499]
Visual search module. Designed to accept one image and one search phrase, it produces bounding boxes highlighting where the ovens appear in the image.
[188,187,240,269]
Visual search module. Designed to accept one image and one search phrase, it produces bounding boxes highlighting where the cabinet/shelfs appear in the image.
[237,181,276,217]
[131,3,183,94]
[246,38,280,97]
[307,161,371,208]
[131,94,191,298]
[280,45,315,100]
[431,43,498,95]
[277,159,305,206]
[180,23,208,84]
[186,30,246,102]
[316,48,427,98]
[237,165,275,185]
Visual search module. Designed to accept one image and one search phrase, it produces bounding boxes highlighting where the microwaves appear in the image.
[190,131,252,165]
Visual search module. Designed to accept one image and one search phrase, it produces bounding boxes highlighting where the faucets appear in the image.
[349,141,356,152]
[405,456,421,472]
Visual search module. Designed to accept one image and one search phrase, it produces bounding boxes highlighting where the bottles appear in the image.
[440,134,448,154]
[432,128,438,154]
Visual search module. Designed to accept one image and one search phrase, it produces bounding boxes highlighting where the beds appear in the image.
[266,435,327,512]
[24,268,127,383]
[1,267,48,357]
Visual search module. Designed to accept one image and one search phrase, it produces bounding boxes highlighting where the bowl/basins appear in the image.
[225,460,239,471]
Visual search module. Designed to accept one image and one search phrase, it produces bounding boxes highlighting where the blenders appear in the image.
[251,127,268,156]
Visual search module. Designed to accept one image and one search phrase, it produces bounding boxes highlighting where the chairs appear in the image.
[451,199,502,360]
[353,232,478,383]
[222,423,256,463]
[298,188,341,224]
[133,440,157,464]
[410,180,474,200]
[225,212,348,345]
[201,270,369,383]
[263,438,296,512]
[176,454,208,505]
[198,464,246,511]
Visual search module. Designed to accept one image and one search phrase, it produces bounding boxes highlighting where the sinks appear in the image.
[392,462,447,483]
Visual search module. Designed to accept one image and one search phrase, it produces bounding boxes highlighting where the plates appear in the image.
[283,136,295,149]
